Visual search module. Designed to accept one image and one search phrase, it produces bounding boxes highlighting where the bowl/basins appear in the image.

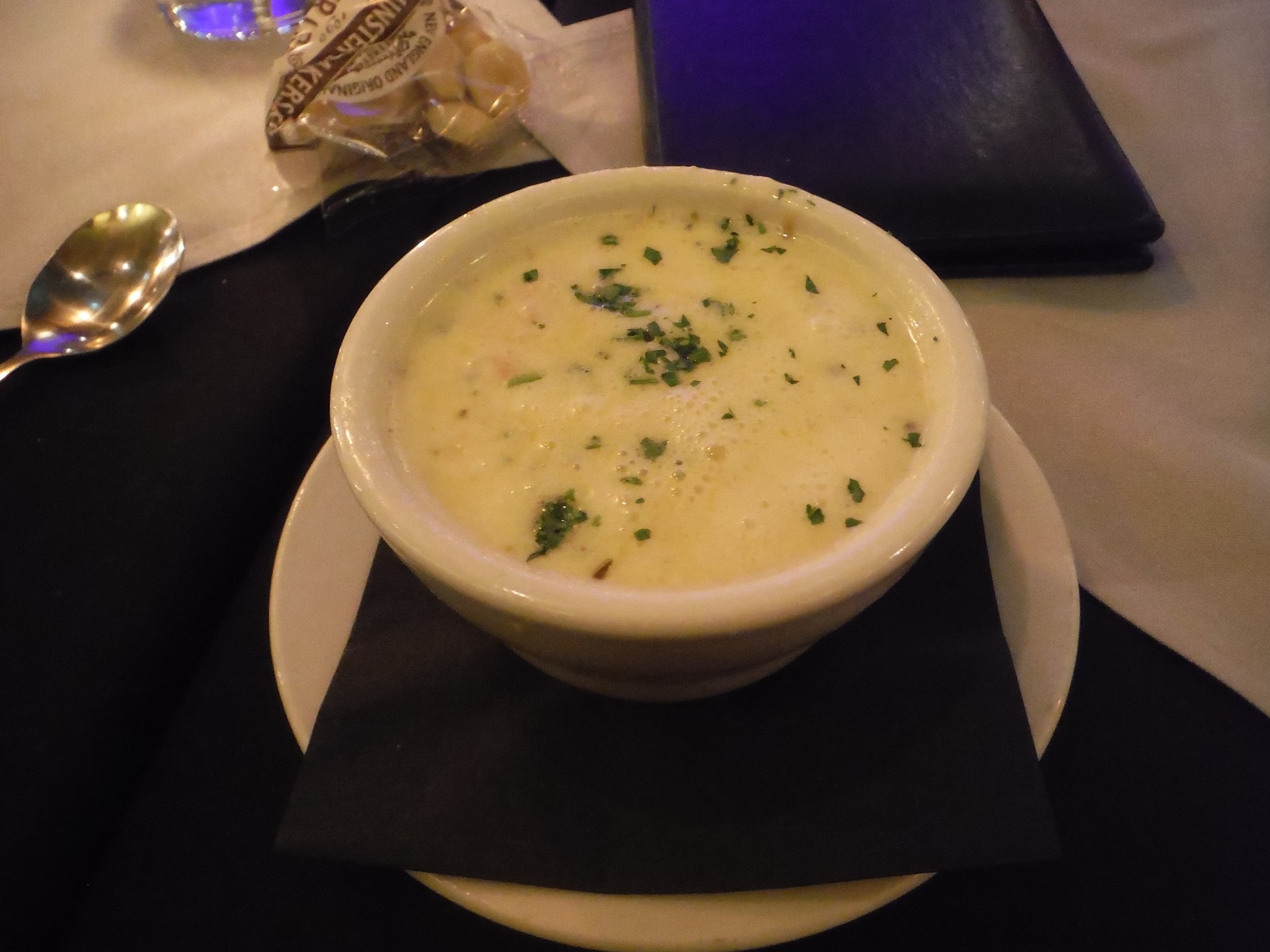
[331,166,989,701]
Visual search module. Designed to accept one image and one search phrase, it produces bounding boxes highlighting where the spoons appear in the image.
[0,203,184,381]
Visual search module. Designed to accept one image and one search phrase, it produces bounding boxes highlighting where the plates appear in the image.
[267,398,1080,952]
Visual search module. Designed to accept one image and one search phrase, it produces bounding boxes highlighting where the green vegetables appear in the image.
[506,216,923,564]
